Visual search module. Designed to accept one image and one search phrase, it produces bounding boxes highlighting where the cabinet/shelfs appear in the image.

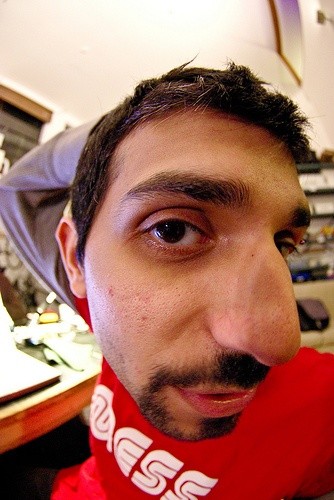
[276,154,334,280]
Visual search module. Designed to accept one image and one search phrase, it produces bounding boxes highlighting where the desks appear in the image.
[0,320,104,453]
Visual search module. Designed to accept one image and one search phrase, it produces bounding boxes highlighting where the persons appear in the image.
[0,53,334,500]
